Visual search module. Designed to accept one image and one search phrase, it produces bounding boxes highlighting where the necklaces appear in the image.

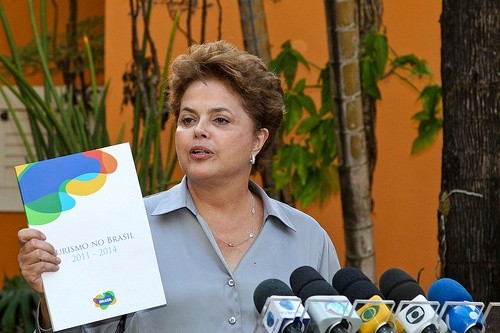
[213,191,256,247]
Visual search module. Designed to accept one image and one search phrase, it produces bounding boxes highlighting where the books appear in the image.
[14,141,167,333]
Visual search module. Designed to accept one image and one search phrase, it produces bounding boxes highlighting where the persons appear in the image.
[17,40,342,333]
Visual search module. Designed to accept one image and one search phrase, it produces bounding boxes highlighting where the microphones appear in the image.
[428,279,487,333]
[379,268,448,332]
[333,267,404,332]
[289,266,360,332]
[254,278,312,332]
